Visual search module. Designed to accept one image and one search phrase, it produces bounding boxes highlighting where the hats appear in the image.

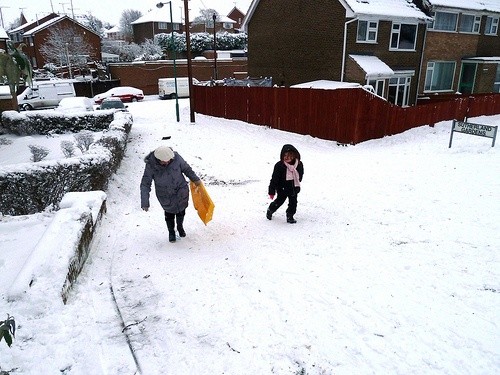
[154,144,175,161]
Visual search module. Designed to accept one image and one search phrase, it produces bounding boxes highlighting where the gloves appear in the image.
[142,207,148,211]
[270,195,274,199]
[195,180,201,185]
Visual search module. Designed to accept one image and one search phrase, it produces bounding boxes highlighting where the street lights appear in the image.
[156,0,180,122]
[212,12,217,80]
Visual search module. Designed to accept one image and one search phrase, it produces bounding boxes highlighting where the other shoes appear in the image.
[177,225,186,237]
[267,209,273,220]
[169,230,176,243]
[287,216,296,223]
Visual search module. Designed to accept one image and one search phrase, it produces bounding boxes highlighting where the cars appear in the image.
[54,97,96,113]
[95,97,129,113]
[93,86,144,105]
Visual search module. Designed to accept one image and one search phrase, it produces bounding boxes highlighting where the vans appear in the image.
[158,77,201,100]
[17,83,76,110]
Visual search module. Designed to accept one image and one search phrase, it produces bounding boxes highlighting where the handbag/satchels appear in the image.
[190,179,215,226]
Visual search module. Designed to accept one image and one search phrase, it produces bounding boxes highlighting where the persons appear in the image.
[265,144,304,224]
[139,146,200,243]
